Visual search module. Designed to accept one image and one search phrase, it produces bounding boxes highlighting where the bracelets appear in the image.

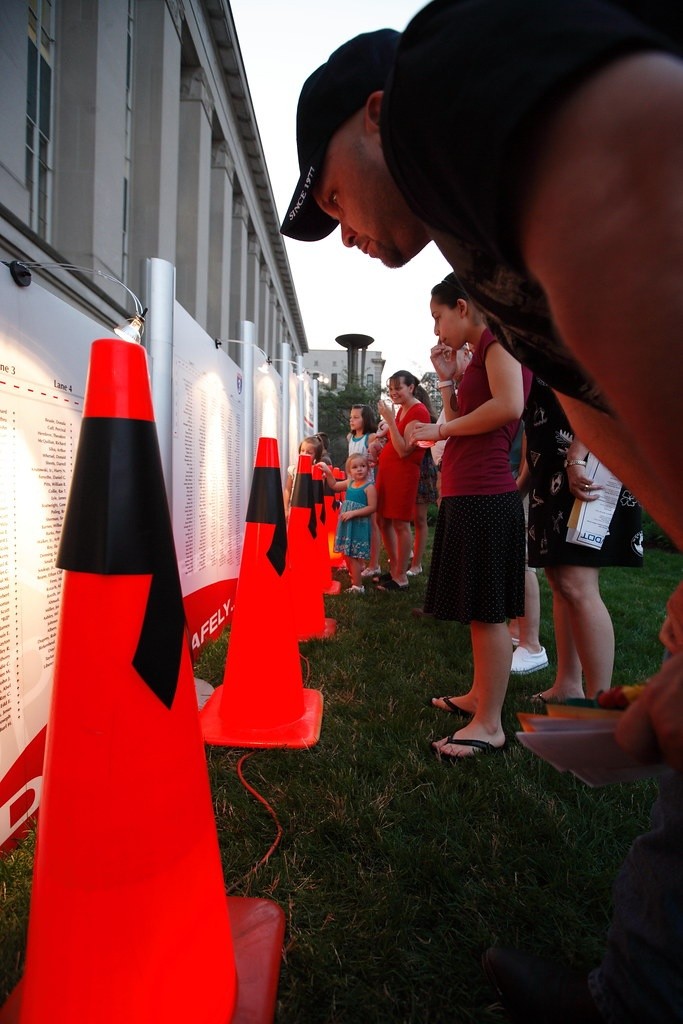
[439,423,448,440]
[434,381,454,390]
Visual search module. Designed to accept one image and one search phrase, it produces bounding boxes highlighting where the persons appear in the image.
[282,432,332,527]
[406,272,528,755]
[274,0,683,773]
[528,367,646,705]
[316,452,377,597]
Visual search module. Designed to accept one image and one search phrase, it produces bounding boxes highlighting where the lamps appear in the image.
[270,359,329,384]
[10,260,148,345]
[215,339,273,374]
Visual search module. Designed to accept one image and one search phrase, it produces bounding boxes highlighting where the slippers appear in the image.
[373,572,393,583]
[376,580,410,591]
[429,696,505,721]
[522,691,549,705]
[430,733,505,759]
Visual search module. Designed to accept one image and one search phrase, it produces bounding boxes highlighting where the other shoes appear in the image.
[349,563,366,576]
[387,549,414,563]
[344,585,365,593]
[361,565,381,576]
[406,565,422,575]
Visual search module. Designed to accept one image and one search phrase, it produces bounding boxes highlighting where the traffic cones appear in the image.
[311,463,345,596]
[287,454,337,642]
[0,338,284,1024]
[197,438,325,750]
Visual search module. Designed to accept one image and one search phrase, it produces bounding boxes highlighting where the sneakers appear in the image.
[510,646,549,674]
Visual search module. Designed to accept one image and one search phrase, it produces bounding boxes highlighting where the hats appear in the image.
[281,29,401,242]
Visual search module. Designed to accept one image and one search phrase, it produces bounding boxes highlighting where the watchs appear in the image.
[564,459,586,468]
